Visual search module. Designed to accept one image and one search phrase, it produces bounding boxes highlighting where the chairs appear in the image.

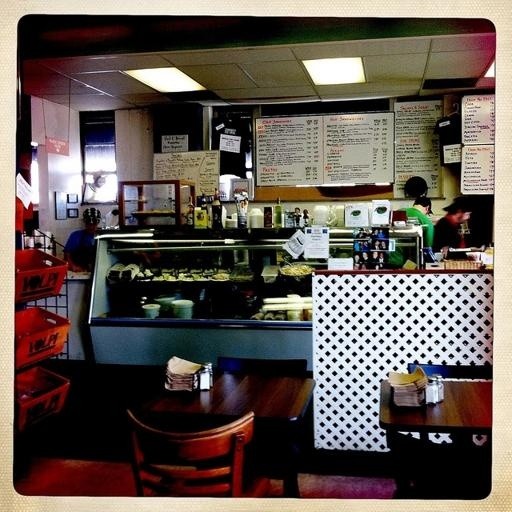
[407,362,491,378]
[125,409,255,496]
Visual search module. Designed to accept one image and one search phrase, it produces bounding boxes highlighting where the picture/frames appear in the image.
[67,193,78,218]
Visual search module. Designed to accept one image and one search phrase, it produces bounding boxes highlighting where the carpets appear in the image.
[19,457,394,497]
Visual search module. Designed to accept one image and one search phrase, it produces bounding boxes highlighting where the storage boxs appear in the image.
[14,249,72,436]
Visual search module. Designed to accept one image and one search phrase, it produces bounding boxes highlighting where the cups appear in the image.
[312,205,336,226]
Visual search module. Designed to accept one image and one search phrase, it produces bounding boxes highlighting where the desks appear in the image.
[140,369,315,498]
[378,379,491,496]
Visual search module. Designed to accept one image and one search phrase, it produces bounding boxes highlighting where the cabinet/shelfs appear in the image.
[87,230,313,371]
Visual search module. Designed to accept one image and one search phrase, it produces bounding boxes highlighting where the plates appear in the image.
[280,263,313,276]
[151,278,230,282]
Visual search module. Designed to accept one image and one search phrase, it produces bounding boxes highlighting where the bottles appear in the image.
[201,192,207,214]
[198,361,214,392]
[425,373,445,407]
[485,242,494,269]
[211,187,224,229]
[273,195,283,229]
[186,196,195,228]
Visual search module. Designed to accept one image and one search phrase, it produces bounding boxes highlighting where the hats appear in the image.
[415,197,434,215]
[441,197,474,213]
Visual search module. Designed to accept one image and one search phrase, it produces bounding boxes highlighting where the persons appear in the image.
[385,195,434,269]
[434,194,483,261]
[64,207,106,273]
